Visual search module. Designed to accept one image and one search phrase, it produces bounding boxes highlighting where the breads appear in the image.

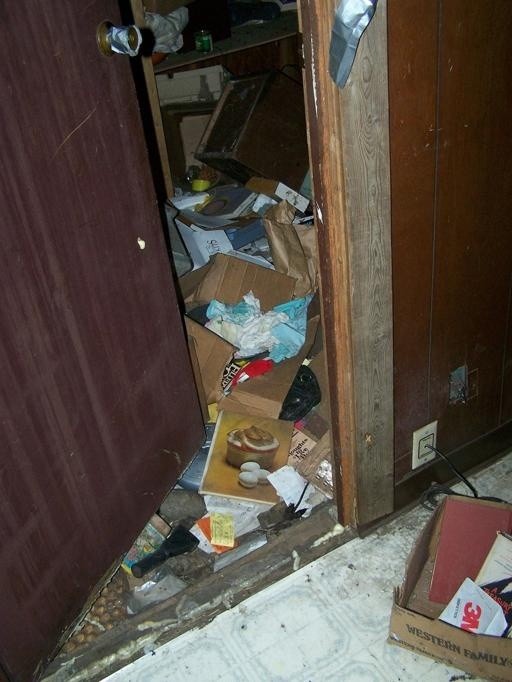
[226,425,279,469]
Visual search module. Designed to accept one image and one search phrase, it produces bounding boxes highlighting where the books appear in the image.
[426,494,511,604]
[473,530,511,639]
[196,410,295,507]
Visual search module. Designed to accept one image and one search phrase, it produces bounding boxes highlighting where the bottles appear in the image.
[195,31,213,53]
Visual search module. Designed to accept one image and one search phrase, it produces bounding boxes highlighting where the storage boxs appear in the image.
[386,496,512,682]
[172,184,322,423]
[193,70,309,196]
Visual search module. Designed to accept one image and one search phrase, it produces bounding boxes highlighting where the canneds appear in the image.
[194,28,214,53]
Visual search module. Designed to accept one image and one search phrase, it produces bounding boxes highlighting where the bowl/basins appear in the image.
[152,52,167,64]
[191,179,211,191]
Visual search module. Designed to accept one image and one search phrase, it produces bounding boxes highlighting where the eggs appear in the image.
[239,461,271,489]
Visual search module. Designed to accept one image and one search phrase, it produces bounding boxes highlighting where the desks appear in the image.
[153,0,313,192]
